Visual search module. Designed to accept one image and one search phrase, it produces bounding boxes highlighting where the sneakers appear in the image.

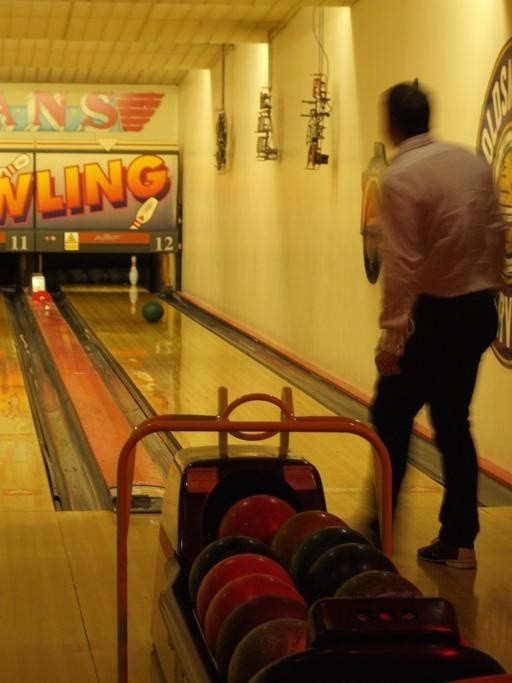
[418,538,478,569]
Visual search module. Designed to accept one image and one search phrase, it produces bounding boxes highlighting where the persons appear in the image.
[370,82,506,569]
